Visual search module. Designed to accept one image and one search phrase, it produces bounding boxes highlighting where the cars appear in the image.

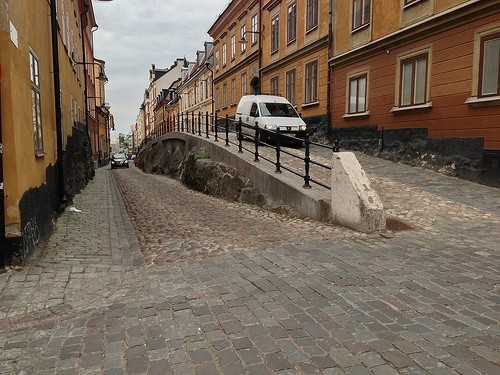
[109,153,129,169]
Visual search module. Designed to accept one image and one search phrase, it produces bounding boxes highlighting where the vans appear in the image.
[234,94,307,149]
